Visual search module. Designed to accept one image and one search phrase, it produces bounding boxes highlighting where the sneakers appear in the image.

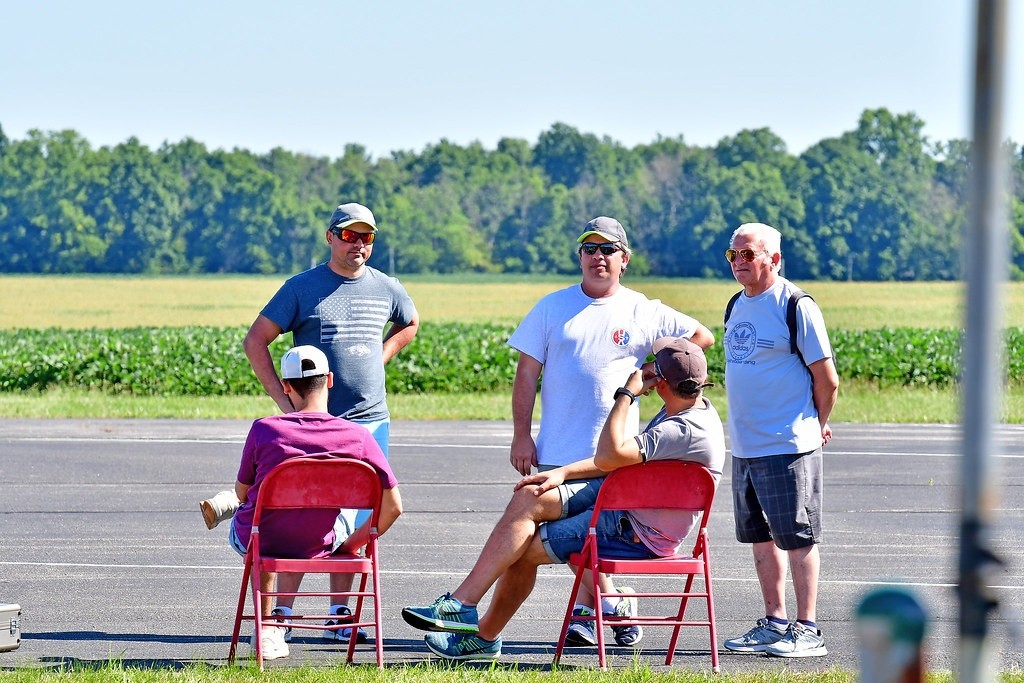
[565,605,597,645]
[402,592,480,633]
[602,586,643,646]
[272,608,292,641]
[766,622,828,657]
[250,627,290,660]
[199,491,233,530]
[424,631,502,660]
[322,607,367,641]
[724,618,788,651]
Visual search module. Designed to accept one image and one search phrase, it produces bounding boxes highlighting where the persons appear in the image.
[242,202,419,642]
[505,216,716,646]
[723,222,839,658]
[400,338,726,661]
[200,346,403,661]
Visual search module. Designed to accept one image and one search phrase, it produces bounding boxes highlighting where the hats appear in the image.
[280,344,330,378]
[577,216,629,253]
[327,203,378,231]
[652,336,715,394]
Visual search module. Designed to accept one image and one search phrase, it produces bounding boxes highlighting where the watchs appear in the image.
[613,387,635,405]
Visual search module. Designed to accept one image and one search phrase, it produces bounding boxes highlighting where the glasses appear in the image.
[652,361,669,381]
[330,227,375,245]
[725,248,773,262]
[578,242,626,255]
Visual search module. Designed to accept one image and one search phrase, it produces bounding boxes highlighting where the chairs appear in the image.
[548,459,720,678]
[227,459,386,672]
